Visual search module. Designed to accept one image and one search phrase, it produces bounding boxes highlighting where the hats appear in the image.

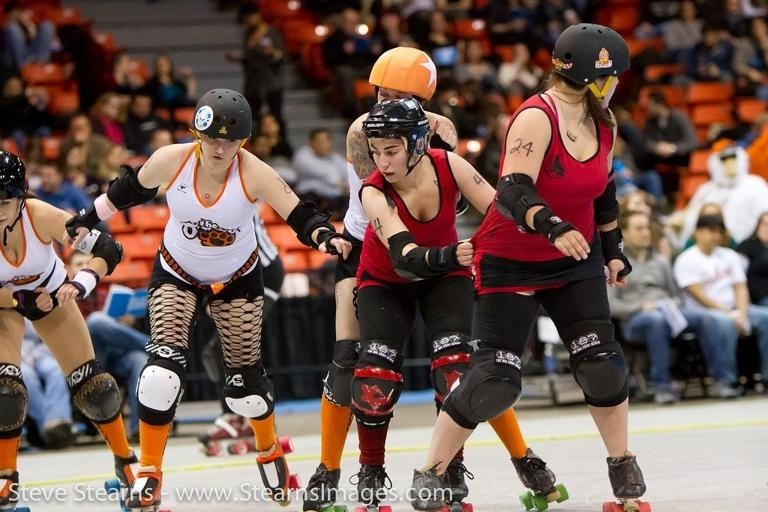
[697,214,727,231]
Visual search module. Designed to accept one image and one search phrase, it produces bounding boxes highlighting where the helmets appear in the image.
[362,97,433,169]
[1,151,29,199]
[368,45,437,101]
[552,23,632,86]
[192,89,253,139]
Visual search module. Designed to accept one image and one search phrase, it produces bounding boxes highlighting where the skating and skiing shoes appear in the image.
[0,470,19,509]
[604,455,653,512]
[349,463,392,512]
[412,456,475,512]
[105,449,162,511]
[199,413,255,455]
[256,436,301,506]
[302,464,348,512]
[510,447,568,512]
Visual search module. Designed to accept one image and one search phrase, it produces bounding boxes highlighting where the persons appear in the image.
[0,1,766,451]
[302,46,557,512]
[63,87,351,512]
[1,149,140,512]
[408,23,644,511]
[349,99,498,512]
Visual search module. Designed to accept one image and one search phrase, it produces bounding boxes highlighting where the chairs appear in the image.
[616,318,768,400]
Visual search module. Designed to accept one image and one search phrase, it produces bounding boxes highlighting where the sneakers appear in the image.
[654,390,675,404]
[43,417,76,447]
[709,382,735,397]
